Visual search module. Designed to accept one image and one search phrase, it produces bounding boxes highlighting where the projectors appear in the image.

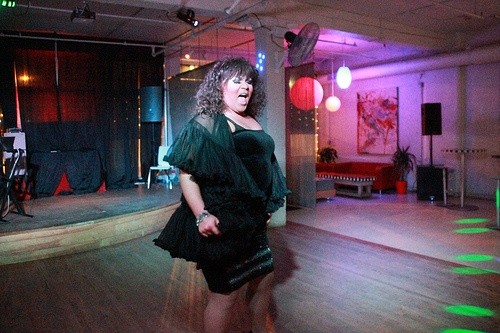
[71,10,95,24]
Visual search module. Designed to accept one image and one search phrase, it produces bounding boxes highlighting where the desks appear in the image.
[441,148,486,211]
[316,174,377,199]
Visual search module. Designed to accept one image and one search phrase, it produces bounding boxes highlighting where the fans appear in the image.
[285,22,320,66]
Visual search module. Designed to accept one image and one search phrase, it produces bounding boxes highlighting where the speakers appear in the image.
[140,86,163,122]
[421,103,442,135]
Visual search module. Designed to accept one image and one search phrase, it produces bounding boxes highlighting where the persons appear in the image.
[153,56,287,333]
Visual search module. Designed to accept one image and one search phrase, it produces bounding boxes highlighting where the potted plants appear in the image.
[392,145,417,194]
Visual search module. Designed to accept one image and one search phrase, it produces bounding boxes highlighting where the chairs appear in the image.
[147,145,174,190]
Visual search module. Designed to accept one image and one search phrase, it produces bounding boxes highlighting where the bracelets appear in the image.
[195,211,209,226]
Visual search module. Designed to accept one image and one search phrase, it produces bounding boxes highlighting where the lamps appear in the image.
[141,85,163,183]
[177,8,199,27]
[336,54,352,89]
[324,55,341,112]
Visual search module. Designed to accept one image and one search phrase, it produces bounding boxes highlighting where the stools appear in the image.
[435,165,456,207]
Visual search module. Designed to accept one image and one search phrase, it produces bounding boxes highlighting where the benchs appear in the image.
[316,161,400,200]
